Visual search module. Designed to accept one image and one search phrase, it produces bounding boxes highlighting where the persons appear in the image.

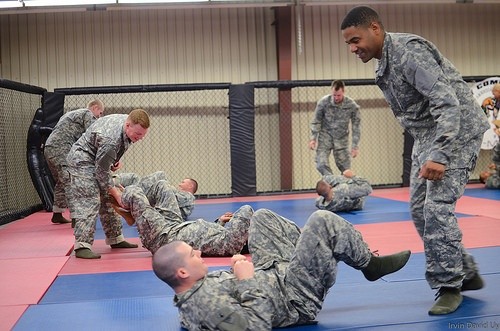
[111,170,198,221]
[152,208,411,331]
[478,82,500,190]
[310,80,361,175]
[314,169,372,214]
[111,185,255,257]
[340,6,490,315]
[62,109,151,259]
[44,99,104,223]
